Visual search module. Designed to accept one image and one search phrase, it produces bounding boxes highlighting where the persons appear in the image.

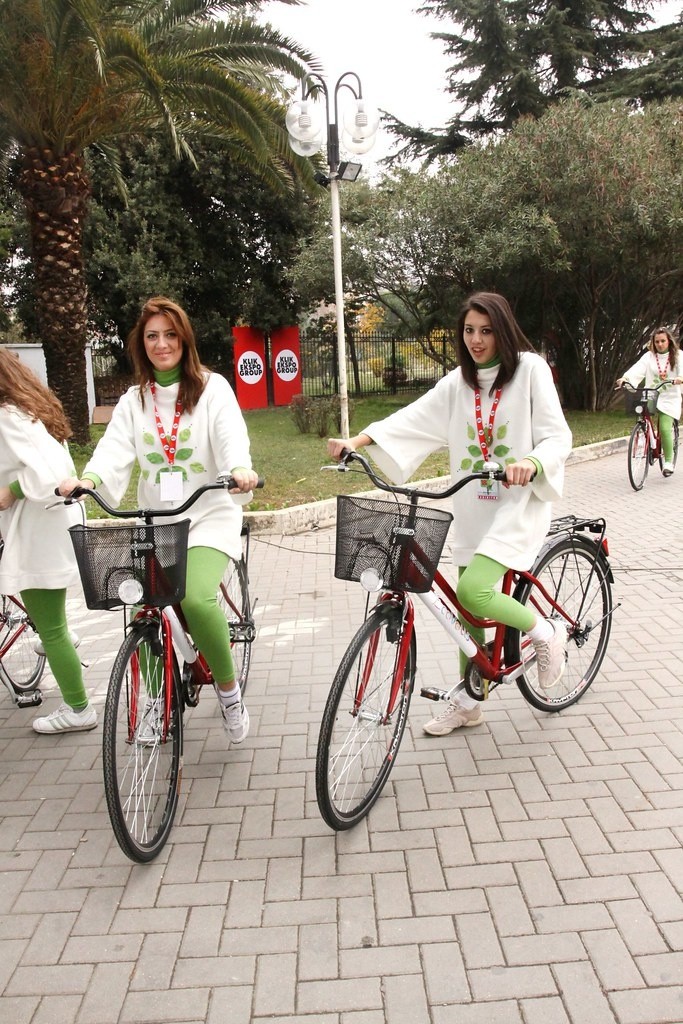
[327,292,573,736]
[60,296,258,744]
[617,328,683,473]
[0,346,97,734]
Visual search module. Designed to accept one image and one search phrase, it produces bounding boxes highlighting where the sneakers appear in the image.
[214,679,250,743]
[636,437,657,449]
[662,461,674,477]
[533,619,568,689]
[423,700,484,735]
[33,701,98,734]
[138,719,176,747]
[34,629,81,656]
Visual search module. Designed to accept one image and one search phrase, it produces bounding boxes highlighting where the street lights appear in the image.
[285,71,381,439]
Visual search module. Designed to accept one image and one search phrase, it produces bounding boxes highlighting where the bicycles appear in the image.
[622,381,683,491]
[0,471,265,863]
[315,448,621,832]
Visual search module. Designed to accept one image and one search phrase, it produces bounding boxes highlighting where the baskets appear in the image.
[334,495,454,593]
[624,388,660,416]
[67,518,191,610]
